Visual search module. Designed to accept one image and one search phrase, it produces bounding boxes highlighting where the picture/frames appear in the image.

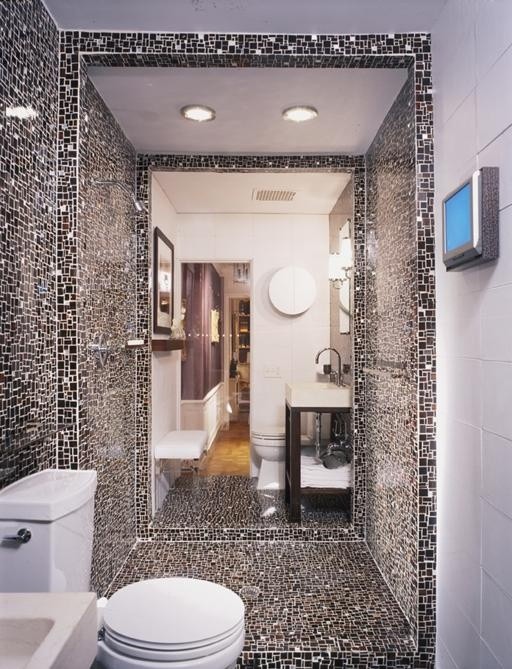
[154,227,174,334]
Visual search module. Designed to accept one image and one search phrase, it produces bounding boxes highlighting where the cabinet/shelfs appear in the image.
[233,313,250,364]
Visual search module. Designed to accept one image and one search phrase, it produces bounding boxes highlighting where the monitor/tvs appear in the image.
[442,169,482,268]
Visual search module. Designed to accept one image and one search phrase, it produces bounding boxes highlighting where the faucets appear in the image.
[315,347,342,386]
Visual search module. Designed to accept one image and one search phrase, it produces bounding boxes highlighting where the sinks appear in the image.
[285,379,350,407]
[0,591,99,669]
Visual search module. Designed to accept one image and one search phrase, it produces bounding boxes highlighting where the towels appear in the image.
[300,447,352,490]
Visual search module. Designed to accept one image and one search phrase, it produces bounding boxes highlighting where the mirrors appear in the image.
[139,154,367,542]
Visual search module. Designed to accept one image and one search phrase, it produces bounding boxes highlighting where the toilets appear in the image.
[250,412,331,491]
[0,470,246,669]
